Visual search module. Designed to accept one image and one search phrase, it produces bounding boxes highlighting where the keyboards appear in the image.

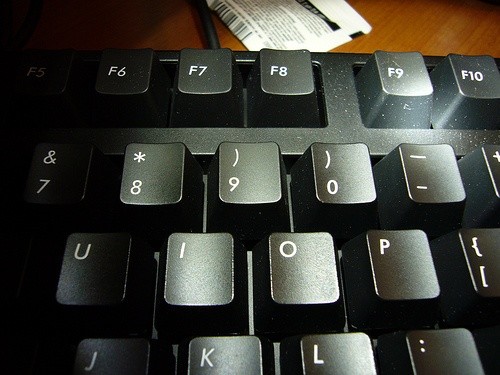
[0,46,500,375]
